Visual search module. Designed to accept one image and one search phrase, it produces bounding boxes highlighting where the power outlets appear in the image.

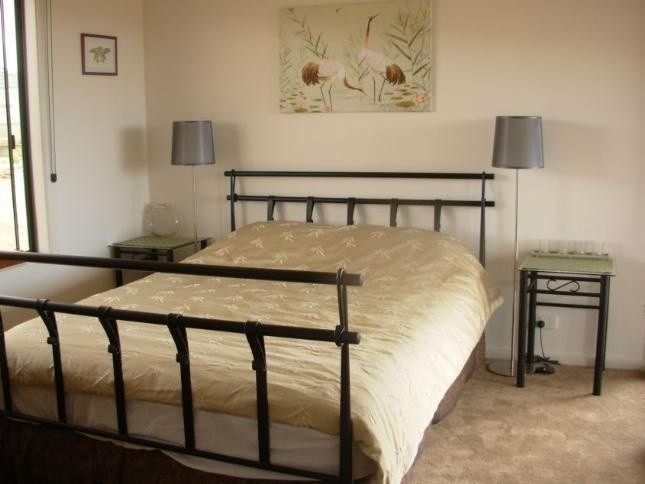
[535,310,559,329]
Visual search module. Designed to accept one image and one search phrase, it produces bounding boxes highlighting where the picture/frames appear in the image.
[81,32,118,75]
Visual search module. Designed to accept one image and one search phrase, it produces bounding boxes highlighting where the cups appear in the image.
[549,241,560,254]
[599,243,608,256]
[583,242,594,255]
[567,240,577,254]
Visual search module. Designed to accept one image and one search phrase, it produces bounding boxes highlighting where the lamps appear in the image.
[171,120,216,253]
[487,116,544,377]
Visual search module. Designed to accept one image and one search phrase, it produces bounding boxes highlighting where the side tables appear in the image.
[107,235,212,287]
[515,252,615,395]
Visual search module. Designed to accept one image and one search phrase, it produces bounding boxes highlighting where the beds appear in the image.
[0,170,505,484]
